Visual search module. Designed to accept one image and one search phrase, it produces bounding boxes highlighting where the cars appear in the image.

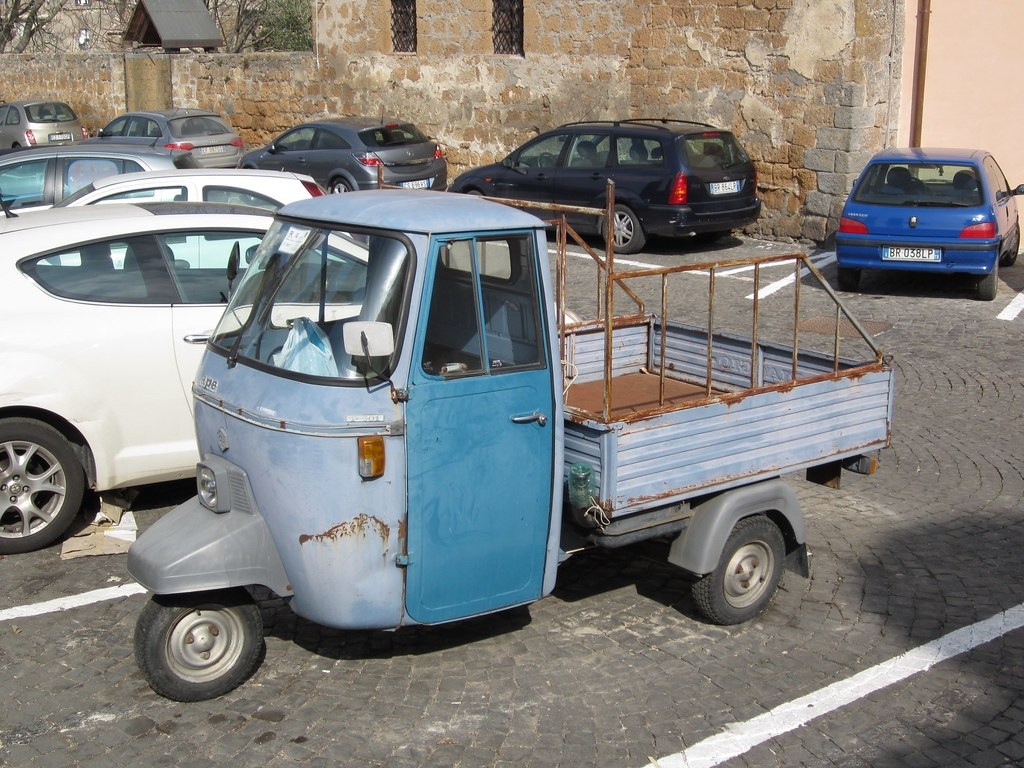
[83,108,242,169]
[0,144,204,217]
[0,202,578,553]
[49,169,327,214]
[239,117,448,194]
[0,100,84,153]
[834,147,1024,301]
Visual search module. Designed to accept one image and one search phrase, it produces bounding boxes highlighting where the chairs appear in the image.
[123,241,176,284]
[627,144,649,163]
[887,166,922,196]
[40,109,52,120]
[689,141,725,165]
[948,170,979,199]
[364,131,405,144]
[573,140,601,167]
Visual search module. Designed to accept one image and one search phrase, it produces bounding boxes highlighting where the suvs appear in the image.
[447,117,761,254]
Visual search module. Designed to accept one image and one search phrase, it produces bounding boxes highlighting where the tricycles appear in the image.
[128,175,894,703]
